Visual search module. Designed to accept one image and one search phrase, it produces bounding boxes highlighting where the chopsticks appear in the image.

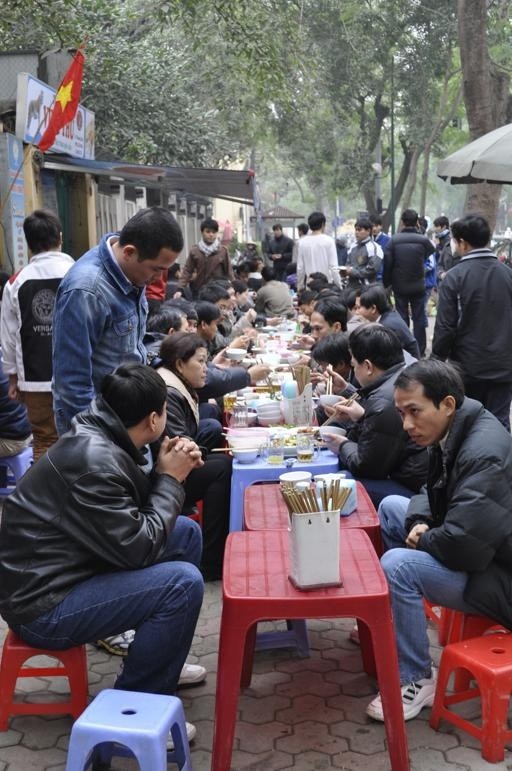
[288,363,311,394]
[278,479,353,525]
[320,393,360,427]
[211,446,268,451]
[256,358,276,393]
[327,363,333,395]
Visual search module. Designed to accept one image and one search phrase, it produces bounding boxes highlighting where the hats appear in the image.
[160,295,199,320]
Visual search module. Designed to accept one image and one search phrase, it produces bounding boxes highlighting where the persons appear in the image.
[166,303,197,335]
[298,290,320,333]
[261,232,272,266]
[240,240,259,262]
[355,284,422,359]
[211,279,257,351]
[346,291,355,316]
[285,262,299,288]
[212,280,257,338]
[146,307,273,454]
[337,235,348,266]
[254,266,294,317]
[434,217,451,253]
[232,281,281,328]
[306,334,361,398]
[266,224,294,272]
[437,240,461,279]
[417,217,436,328]
[432,216,512,430]
[292,301,349,372]
[198,285,232,349]
[237,262,260,291]
[292,223,308,264]
[324,326,431,506]
[0,209,73,464]
[191,299,257,361]
[341,218,383,287]
[150,331,233,582]
[51,207,183,657]
[172,219,235,300]
[382,209,427,360]
[297,213,343,294]
[0,364,33,459]
[298,290,365,351]
[166,263,193,302]
[321,323,419,425]
[369,215,390,284]
[144,269,167,315]
[243,261,263,292]
[255,256,263,272]
[349,358,512,722]
[0,365,207,752]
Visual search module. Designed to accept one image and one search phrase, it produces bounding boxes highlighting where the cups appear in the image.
[225,405,247,430]
[260,435,286,464]
[223,391,238,412]
[297,433,321,463]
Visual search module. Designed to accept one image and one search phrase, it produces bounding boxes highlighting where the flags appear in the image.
[33,47,85,153]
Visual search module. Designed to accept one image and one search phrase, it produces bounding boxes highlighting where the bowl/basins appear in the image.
[288,354,305,367]
[321,426,347,442]
[279,470,312,487]
[320,393,345,409]
[232,447,259,464]
[234,412,258,426]
[226,348,247,363]
[314,473,346,483]
[257,400,282,427]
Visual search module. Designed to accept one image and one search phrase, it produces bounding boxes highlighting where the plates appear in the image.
[271,446,312,458]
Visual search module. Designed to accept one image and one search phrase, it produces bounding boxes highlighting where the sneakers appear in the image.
[177,661,207,687]
[113,722,198,750]
[349,624,360,644]
[89,630,139,656]
[365,667,438,723]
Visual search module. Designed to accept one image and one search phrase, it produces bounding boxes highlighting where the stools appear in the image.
[4,447,34,493]
[1,629,89,731]
[66,689,193,771]
[420,595,511,646]
[227,446,339,661]
[211,528,410,771]
[429,635,512,763]
[190,500,203,525]
[226,316,318,427]
[238,481,380,690]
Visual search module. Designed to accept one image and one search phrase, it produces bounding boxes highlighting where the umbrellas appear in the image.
[436,124,511,185]
